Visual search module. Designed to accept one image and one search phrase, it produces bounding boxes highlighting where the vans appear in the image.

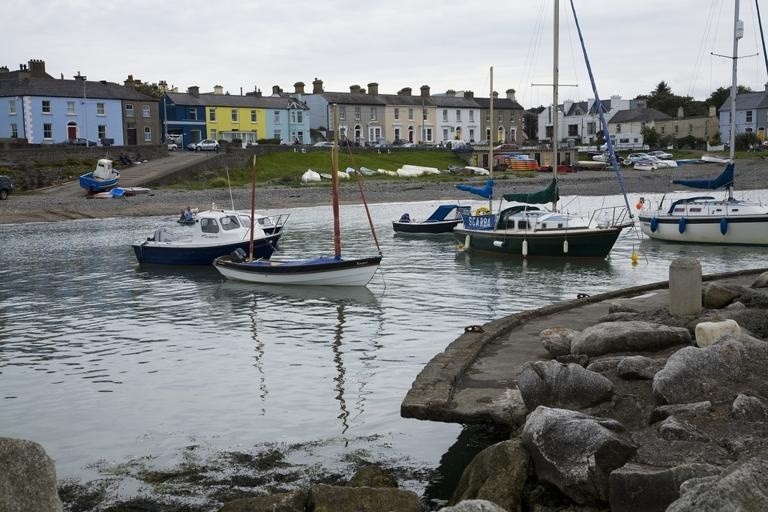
[600,132,644,152]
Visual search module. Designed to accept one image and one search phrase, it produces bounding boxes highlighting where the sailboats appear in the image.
[637,0,768,248]
[392,66,494,237]
[452,0,636,259]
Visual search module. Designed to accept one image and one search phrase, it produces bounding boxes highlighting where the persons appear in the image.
[280,138,287,145]
[119,153,128,165]
[125,153,133,164]
[185,207,192,221]
[179,209,186,221]
[295,138,299,144]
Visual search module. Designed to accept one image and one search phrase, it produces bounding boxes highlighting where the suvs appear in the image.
[186,139,220,151]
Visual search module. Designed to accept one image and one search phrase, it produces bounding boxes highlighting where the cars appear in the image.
[313,141,340,149]
[0,175,14,200]
[59,138,97,147]
[374,142,424,149]
[162,140,179,152]
[495,144,519,150]
[627,150,673,165]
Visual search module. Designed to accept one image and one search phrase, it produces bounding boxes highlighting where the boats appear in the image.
[702,154,731,165]
[633,159,678,171]
[211,248,383,287]
[676,158,705,165]
[78,158,121,192]
[301,166,397,184]
[130,200,291,267]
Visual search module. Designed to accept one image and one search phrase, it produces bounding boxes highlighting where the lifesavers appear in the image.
[476,208,489,216]
